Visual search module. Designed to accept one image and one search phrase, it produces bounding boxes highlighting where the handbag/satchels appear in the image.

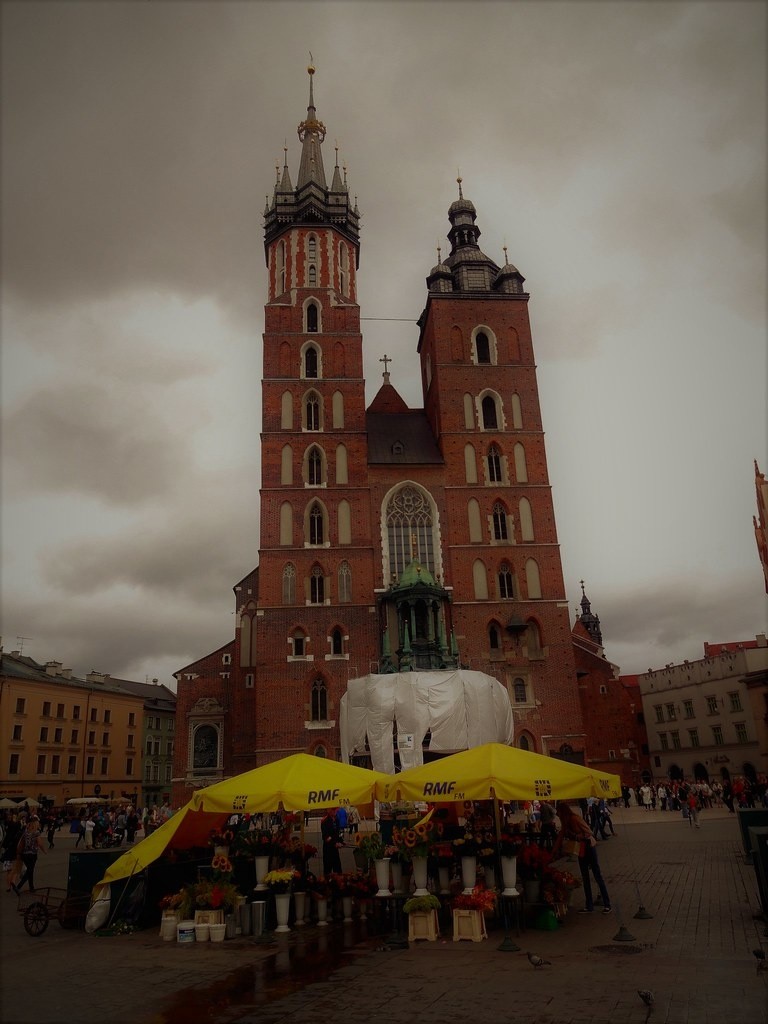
[15,832,27,855]
[562,833,586,857]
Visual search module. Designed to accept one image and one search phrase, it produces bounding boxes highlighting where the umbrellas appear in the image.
[0,797,18,808]
[389,740,624,864]
[17,797,43,808]
[187,751,391,885]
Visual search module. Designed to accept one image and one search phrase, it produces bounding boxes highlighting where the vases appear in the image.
[484,864,497,889]
[374,856,392,897]
[392,861,405,894]
[253,855,270,892]
[438,868,451,895]
[524,880,540,903]
[412,855,430,897]
[294,892,311,926]
[209,923,226,942]
[460,854,476,895]
[500,854,520,897]
[452,908,488,943]
[159,910,187,937]
[226,895,266,939]
[214,846,230,859]
[317,899,329,926]
[194,923,209,941]
[273,894,292,933]
[343,897,354,923]
[552,886,573,919]
[358,901,367,919]
[194,909,224,925]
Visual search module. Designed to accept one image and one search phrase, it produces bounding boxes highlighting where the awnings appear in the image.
[66,795,132,805]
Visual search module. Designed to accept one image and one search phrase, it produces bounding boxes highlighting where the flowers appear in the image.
[499,835,524,859]
[448,886,498,914]
[311,877,330,900]
[263,868,299,894]
[477,843,499,864]
[451,833,484,856]
[392,820,443,859]
[543,870,574,903]
[300,871,316,892]
[384,845,403,862]
[303,844,316,862]
[280,841,303,867]
[159,888,191,916]
[353,832,389,859]
[331,873,355,897]
[521,843,540,881]
[435,845,454,869]
[279,813,297,848]
[211,854,236,881]
[207,828,233,847]
[238,829,279,856]
[182,881,239,910]
[352,880,370,901]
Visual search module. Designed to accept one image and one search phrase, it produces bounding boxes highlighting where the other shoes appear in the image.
[602,837,609,840]
[6,883,15,892]
[29,888,36,892]
[606,833,612,837]
[595,838,600,841]
[14,887,20,896]
[613,833,617,837]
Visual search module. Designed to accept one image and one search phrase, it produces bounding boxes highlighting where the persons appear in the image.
[551,803,613,915]
[224,772,768,879]
[0,800,181,893]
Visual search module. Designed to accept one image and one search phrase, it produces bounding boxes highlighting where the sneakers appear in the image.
[602,907,613,914]
[577,908,595,913]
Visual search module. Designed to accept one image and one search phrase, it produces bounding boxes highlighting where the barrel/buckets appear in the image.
[209,924,226,941]
[162,918,177,941]
[196,925,209,941]
[177,923,195,942]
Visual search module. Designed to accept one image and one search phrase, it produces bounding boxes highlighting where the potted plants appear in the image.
[402,895,443,942]
